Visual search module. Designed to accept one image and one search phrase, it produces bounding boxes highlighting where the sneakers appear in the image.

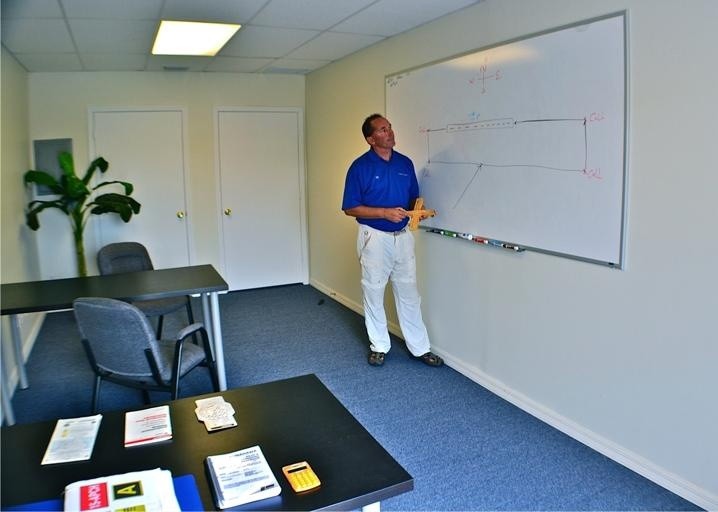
[420,351,444,367]
[367,350,385,366]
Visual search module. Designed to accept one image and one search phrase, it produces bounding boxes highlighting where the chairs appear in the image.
[71,295,225,416]
[97,241,199,345]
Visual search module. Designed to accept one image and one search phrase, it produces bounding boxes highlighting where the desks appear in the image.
[0,374,414,511]
[1,263,241,428]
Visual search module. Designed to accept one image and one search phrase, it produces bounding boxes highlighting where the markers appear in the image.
[426,228,519,251]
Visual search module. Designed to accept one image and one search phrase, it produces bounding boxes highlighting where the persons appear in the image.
[340,113,446,368]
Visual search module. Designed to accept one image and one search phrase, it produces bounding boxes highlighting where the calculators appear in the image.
[282,461,321,493]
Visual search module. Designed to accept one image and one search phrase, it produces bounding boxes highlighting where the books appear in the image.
[123,405,172,448]
[204,443,283,509]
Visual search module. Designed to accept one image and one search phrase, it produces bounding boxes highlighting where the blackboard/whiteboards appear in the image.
[384,7,631,273]
